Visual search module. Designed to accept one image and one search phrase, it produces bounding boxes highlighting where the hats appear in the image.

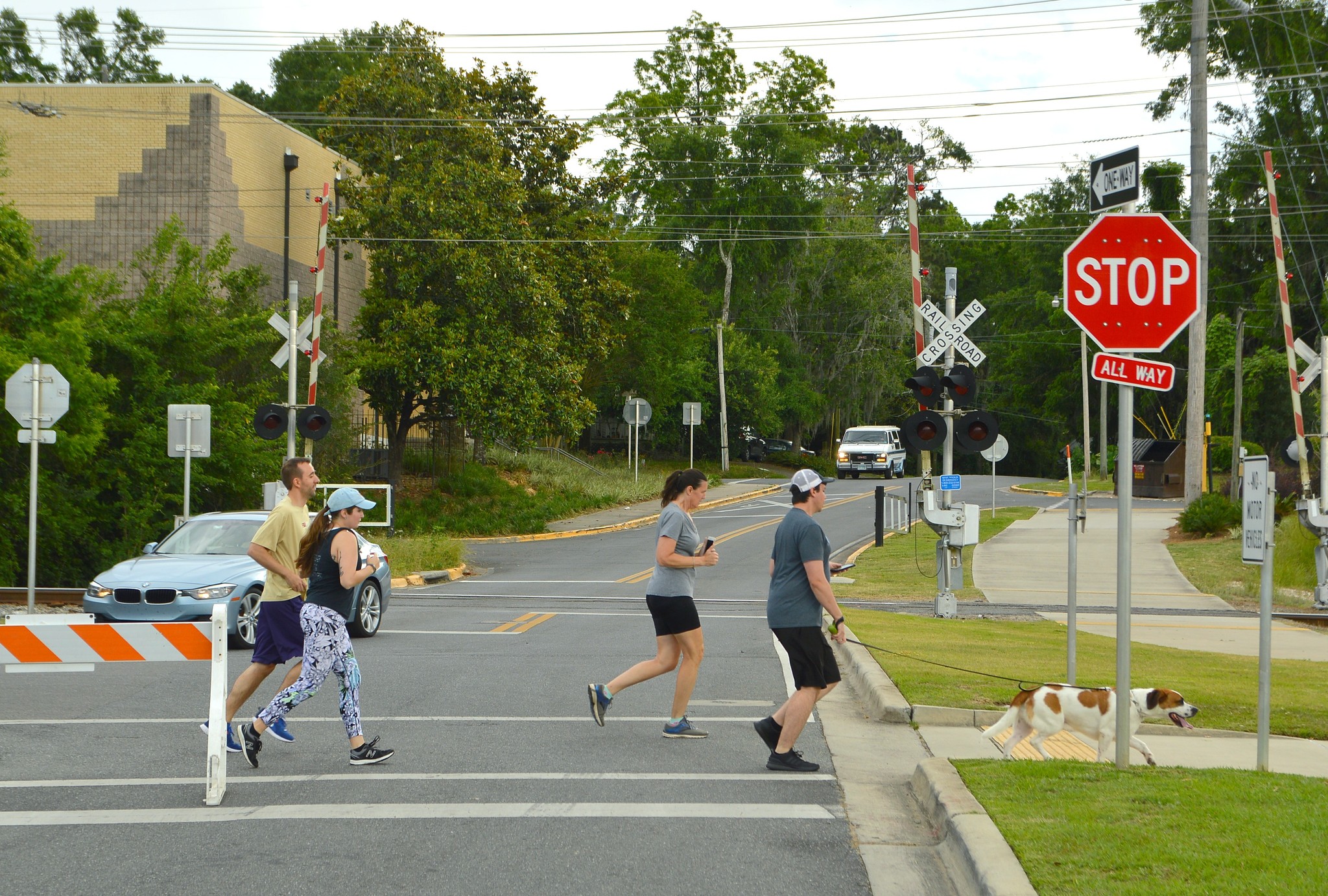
[327,488,376,512]
[788,469,835,492]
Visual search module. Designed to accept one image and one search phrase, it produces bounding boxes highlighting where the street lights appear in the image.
[689,328,730,472]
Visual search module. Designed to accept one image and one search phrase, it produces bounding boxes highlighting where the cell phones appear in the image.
[830,564,856,572]
[705,536,715,554]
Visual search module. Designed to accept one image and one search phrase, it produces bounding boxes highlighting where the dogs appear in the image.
[978,682,1200,768]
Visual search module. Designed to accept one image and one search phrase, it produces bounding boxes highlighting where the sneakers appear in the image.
[766,747,820,771]
[199,719,242,752]
[754,716,793,755]
[662,715,709,738]
[588,683,613,727]
[252,708,296,743]
[350,735,394,765]
[237,723,262,768]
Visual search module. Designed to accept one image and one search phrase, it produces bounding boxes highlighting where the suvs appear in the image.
[711,423,767,463]
[836,426,907,480]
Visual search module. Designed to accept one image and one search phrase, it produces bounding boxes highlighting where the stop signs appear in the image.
[1062,213,1201,353]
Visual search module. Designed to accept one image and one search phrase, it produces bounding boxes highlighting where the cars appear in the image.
[82,509,392,650]
[762,438,816,462]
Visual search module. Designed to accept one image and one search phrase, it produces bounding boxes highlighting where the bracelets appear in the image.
[366,563,376,575]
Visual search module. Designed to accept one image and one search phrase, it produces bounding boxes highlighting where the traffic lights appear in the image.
[907,410,947,451]
[297,406,332,441]
[939,365,976,406]
[904,366,940,408]
[254,404,288,439]
[956,410,999,451]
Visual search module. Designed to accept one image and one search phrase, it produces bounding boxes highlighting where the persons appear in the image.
[588,468,718,738]
[199,457,320,752]
[237,487,395,768]
[752,469,846,772]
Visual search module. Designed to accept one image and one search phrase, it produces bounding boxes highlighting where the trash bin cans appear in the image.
[1113,438,1186,498]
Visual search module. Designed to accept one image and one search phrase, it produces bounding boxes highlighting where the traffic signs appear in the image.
[1089,144,1140,214]
